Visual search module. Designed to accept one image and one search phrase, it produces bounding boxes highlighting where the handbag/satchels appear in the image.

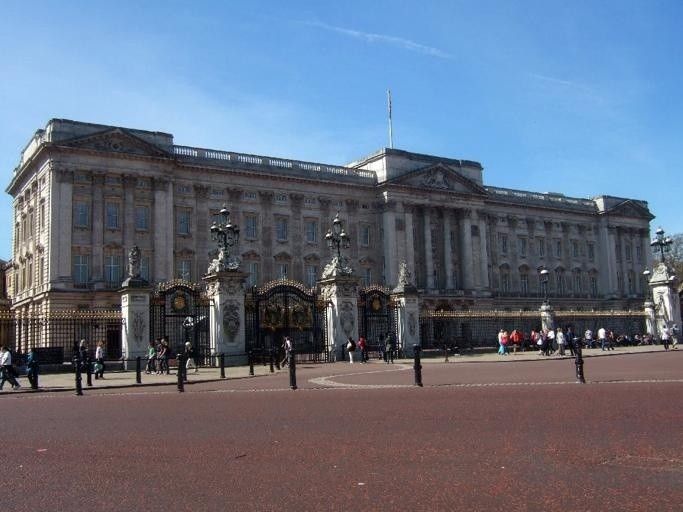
[347,339,352,350]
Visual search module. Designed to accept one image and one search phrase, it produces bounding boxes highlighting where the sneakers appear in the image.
[13,384,21,390]
[143,370,170,376]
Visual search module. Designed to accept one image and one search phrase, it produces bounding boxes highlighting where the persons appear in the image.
[184,341,197,372]
[284,337,293,351]
[497,324,681,356]
[384,333,394,364]
[71,341,81,373]
[25,347,39,389]
[357,336,368,364]
[93,341,106,380]
[346,337,355,365]
[447,335,461,357]
[279,334,295,369]
[78,339,88,372]
[143,337,170,374]
[376,334,385,360]
[0,346,20,390]
